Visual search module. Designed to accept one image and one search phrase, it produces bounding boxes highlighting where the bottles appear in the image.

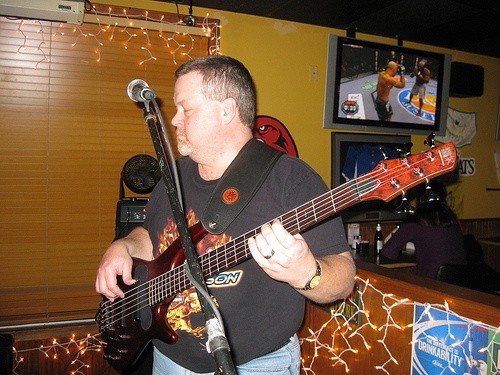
[351,235,358,249]
[358,235,362,245]
[374,221,383,254]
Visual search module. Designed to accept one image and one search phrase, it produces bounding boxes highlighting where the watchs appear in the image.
[291,259,321,291]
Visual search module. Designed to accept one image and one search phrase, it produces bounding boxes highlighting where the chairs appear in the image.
[436,260,486,289]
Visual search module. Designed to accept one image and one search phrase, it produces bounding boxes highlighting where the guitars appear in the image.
[97,134,457,370]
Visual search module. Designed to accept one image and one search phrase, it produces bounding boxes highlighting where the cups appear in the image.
[359,241,369,257]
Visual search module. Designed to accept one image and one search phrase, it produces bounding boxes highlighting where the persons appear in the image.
[404,59,431,116]
[381,180,466,283]
[95,56,356,375]
[372,50,406,121]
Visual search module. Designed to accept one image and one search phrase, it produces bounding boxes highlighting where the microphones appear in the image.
[187,17,195,26]
[126,79,156,103]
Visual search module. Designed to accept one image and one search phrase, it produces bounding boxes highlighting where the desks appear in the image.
[357,246,416,268]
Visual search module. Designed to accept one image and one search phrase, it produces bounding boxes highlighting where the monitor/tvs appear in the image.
[330,131,416,224]
[323,33,452,136]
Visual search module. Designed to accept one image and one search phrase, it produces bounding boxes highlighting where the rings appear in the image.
[264,249,275,259]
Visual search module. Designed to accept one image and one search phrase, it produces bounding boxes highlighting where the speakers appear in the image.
[115,198,151,294]
